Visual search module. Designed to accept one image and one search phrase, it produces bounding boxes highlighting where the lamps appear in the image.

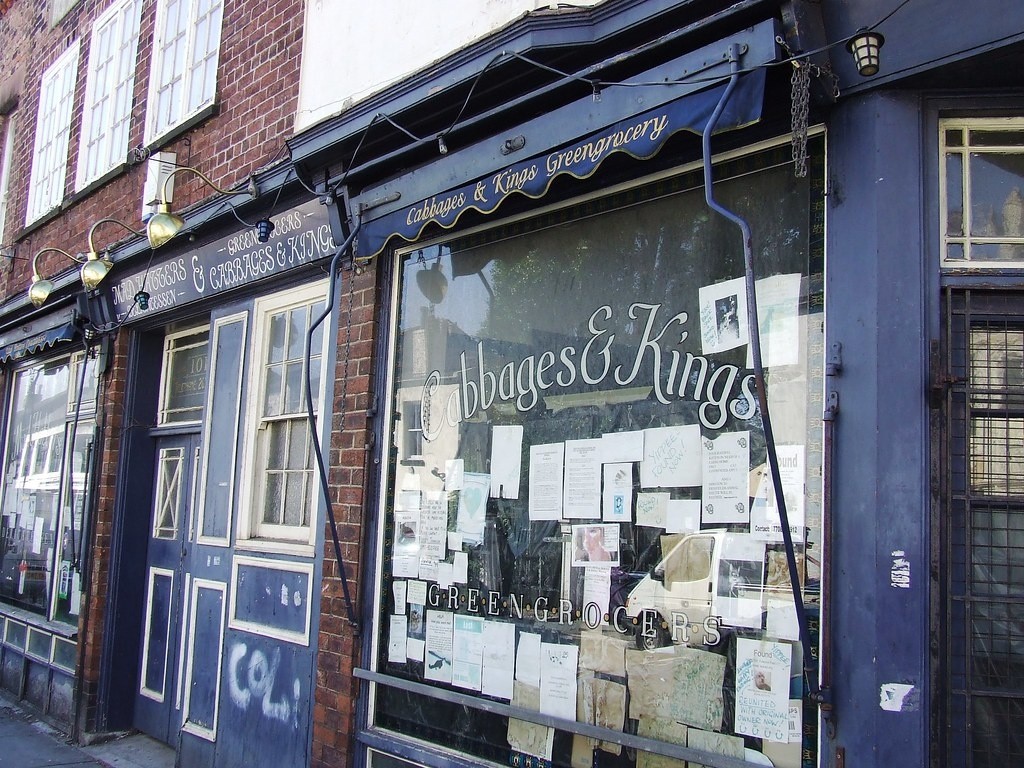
[80,218,148,290]
[845,25,886,76]
[134,291,150,310]
[84,321,95,340]
[147,168,259,252]
[28,248,85,309]
[255,217,274,242]
